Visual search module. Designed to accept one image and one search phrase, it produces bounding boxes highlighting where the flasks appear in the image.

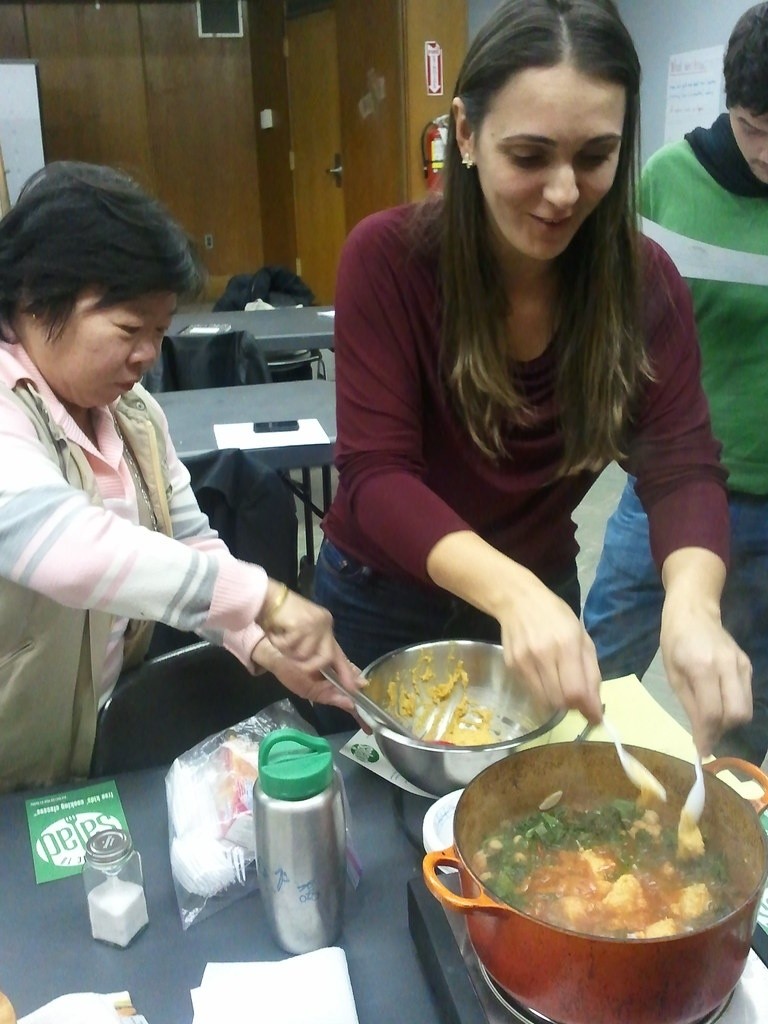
[252,728,352,954]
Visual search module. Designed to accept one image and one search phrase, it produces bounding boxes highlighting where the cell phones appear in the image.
[253,420,299,433]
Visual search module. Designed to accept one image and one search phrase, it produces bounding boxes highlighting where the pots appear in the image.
[420,743,768,1024]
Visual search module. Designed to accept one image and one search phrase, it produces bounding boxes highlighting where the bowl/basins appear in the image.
[356,636,570,800]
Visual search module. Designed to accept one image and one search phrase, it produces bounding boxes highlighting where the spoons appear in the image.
[595,711,671,806]
[677,746,708,833]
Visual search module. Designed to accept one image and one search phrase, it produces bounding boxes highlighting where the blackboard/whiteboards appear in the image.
[0,58,50,217]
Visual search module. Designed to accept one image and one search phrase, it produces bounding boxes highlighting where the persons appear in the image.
[581,1,768,782]
[1,162,374,797]
[306,0,754,757]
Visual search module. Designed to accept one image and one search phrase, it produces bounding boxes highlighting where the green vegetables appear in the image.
[482,795,735,911]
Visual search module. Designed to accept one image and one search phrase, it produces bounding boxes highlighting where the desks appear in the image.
[0,728,440,1023]
[149,381,337,564]
[164,305,335,380]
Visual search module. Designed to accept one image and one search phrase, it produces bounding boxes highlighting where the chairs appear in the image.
[144,330,271,392]
[146,448,298,660]
[213,266,326,382]
[91,642,322,776]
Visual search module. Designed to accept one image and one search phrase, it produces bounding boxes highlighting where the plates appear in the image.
[422,787,465,875]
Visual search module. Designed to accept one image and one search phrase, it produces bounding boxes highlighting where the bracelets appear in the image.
[254,582,289,620]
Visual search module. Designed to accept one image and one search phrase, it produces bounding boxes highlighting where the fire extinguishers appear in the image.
[420,113,451,196]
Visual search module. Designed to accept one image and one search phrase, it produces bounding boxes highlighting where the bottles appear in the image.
[81,830,150,948]
